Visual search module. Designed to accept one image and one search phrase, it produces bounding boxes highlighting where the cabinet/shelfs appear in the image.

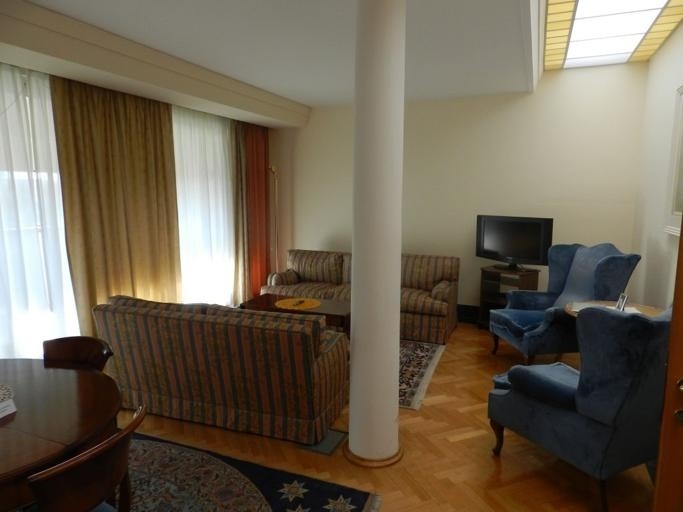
[480,264,539,326]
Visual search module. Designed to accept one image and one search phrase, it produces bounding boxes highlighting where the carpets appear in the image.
[116,427,383,512]
[400,338,447,411]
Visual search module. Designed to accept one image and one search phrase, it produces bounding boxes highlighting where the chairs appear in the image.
[27,336,146,511]
[487,306,674,507]
[488,242,642,371]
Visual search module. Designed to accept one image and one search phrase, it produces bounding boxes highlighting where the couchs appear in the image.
[266,249,463,344]
[92,295,349,446]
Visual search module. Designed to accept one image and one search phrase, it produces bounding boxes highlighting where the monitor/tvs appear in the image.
[474,215,554,273]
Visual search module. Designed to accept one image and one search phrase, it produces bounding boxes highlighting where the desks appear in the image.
[1,358,120,512]
[565,299,665,325]
